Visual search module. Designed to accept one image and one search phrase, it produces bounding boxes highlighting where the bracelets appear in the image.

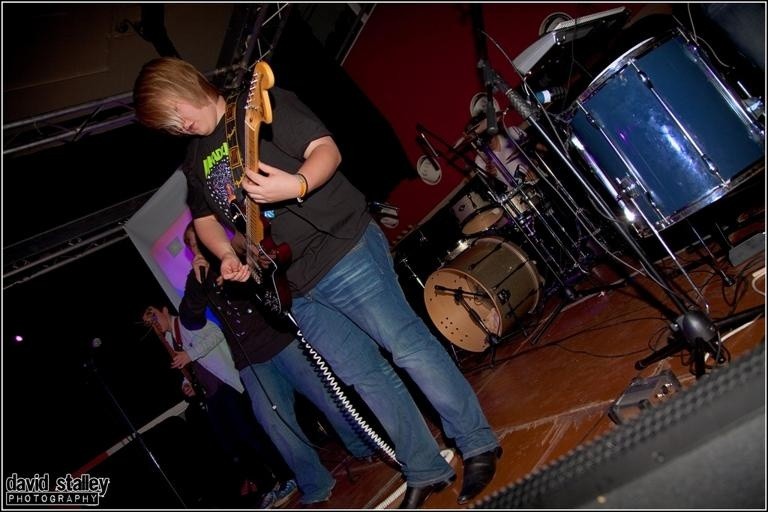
[293,171,309,202]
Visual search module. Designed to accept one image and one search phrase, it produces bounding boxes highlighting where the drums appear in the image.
[451,187,504,236]
[501,178,545,219]
[424,236,541,352]
[554,26,765,239]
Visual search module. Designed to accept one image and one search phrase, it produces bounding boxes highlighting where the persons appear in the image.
[466,116,548,191]
[132,58,502,509]
[177,219,378,502]
[140,300,299,509]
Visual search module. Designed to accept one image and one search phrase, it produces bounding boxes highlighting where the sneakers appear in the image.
[260,478,298,509]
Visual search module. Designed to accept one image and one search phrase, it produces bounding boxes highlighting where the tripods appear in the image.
[453,24,765,379]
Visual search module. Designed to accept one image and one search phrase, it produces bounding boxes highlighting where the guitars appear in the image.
[146,308,209,413]
[224,61,292,314]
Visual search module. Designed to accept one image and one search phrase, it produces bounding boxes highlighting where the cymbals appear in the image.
[452,111,504,150]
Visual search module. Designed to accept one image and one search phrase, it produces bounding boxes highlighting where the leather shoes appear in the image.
[457,447,503,506]
[396,472,456,509]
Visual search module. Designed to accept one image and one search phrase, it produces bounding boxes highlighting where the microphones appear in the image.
[199,266,206,283]
[420,132,439,157]
